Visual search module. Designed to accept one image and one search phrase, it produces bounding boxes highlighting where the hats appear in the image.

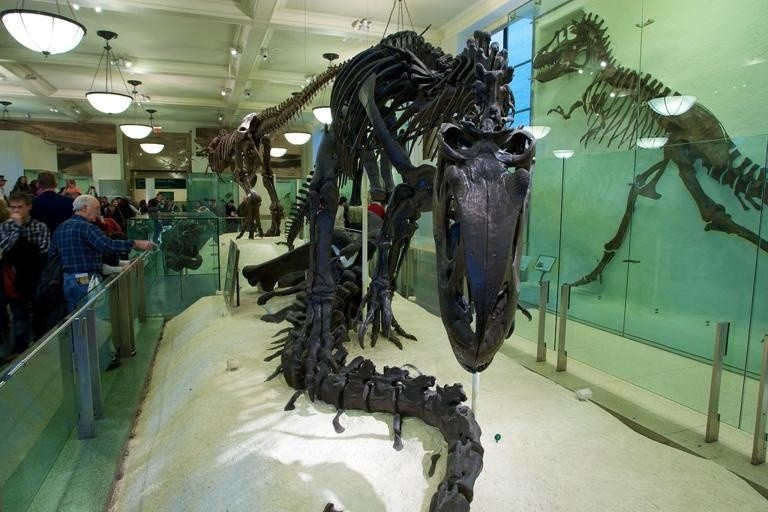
[89,277,105,308]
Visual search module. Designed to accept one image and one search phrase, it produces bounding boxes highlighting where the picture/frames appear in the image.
[532,255,557,272]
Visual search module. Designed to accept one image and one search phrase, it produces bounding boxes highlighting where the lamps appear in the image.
[120,80,153,140]
[229,46,240,56]
[637,137,668,149]
[221,80,234,95]
[312,54,338,125]
[244,89,251,98]
[284,92,311,145]
[110,58,137,71]
[140,110,164,155]
[258,47,268,59]
[554,150,574,159]
[0,0,87,55]
[86,31,133,114]
[646,92,696,117]
[139,94,150,106]
[269,147,287,158]
[521,126,550,139]
[352,18,373,32]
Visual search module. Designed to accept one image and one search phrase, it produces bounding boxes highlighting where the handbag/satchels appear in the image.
[123,218,134,226]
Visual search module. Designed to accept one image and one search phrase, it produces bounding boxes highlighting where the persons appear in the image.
[0,167,236,261]
[333,195,348,232]
[236,195,248,217]
[0,190,50,364]
[46,193,157,374]
[367,196,386,262]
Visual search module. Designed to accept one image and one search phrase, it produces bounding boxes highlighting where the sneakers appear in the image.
[105,356,120,370]
[115,347,136,358]
